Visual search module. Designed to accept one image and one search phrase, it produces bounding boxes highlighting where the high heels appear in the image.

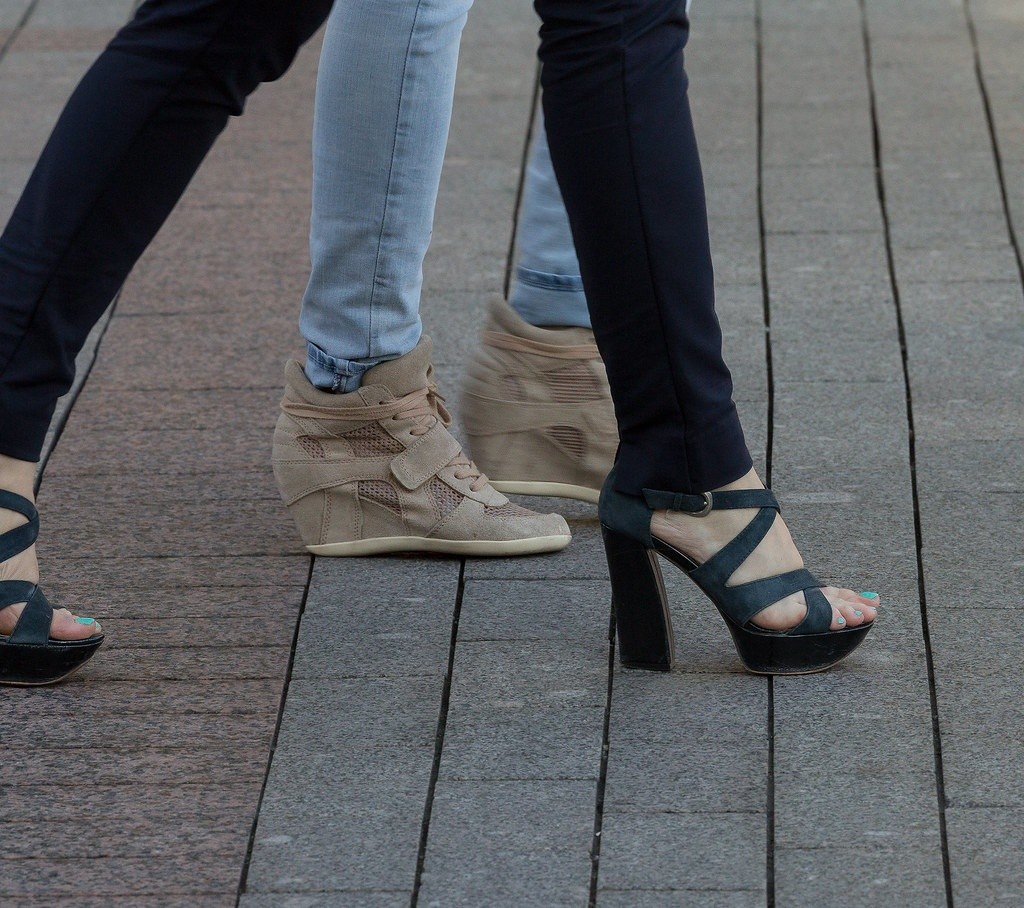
[461,290,622,503]
[0,490,105,686]
[272,335,572,557]
[598,458,875,675]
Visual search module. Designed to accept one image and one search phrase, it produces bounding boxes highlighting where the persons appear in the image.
[1,1,881,687]
[273,0,618,556]
